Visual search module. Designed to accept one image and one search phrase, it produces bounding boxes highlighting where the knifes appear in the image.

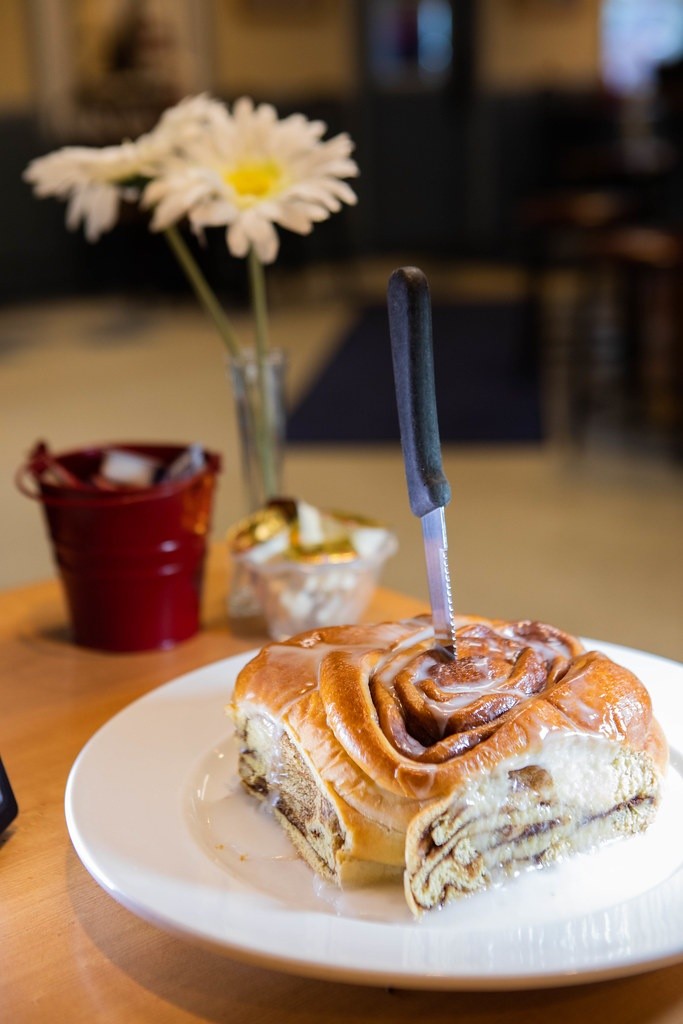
[386,267,457,665]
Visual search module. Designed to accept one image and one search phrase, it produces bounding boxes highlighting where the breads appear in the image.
[231,612,668,915]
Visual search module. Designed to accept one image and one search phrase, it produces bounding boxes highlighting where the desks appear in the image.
[0,539,683,1024]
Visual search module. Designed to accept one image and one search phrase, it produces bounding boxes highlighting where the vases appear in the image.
[230,347,288,511]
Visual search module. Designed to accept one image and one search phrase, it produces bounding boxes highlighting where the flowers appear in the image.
[25,92,359,493]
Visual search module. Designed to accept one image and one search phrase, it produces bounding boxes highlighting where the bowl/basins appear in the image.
[237,524,397,641]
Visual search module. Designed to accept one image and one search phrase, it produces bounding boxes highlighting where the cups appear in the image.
[15,439,223,653]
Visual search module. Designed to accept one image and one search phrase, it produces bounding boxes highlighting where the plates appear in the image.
[63,638,683,987]
